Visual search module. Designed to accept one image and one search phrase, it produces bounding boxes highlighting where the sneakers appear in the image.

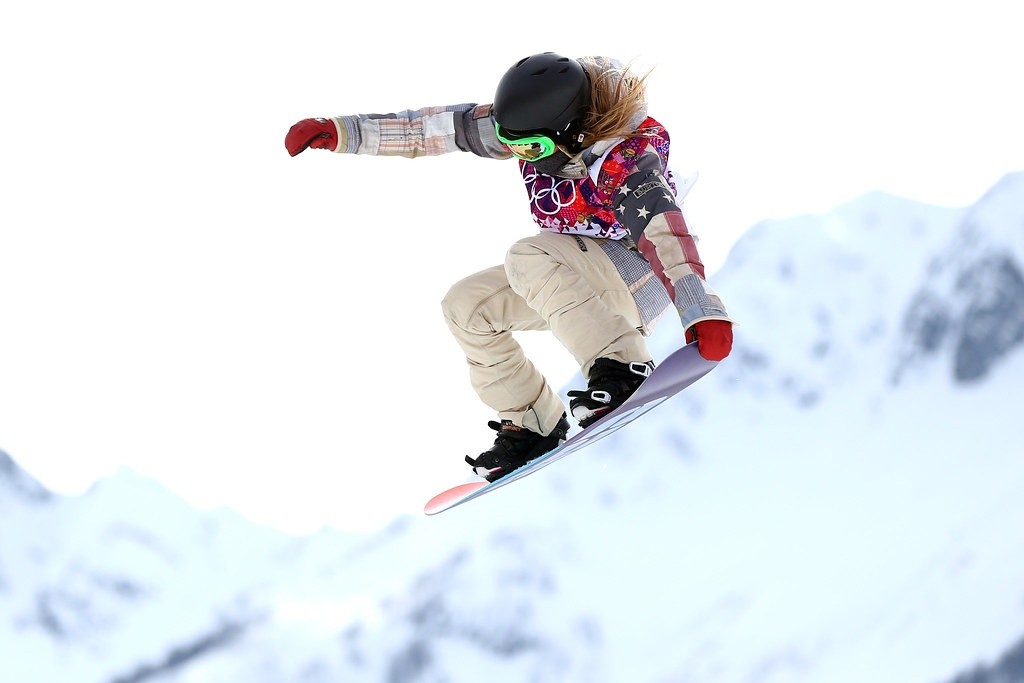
[566,356,664,430]
[464,411,570,484]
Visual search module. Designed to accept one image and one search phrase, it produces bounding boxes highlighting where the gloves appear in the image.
[687,318,734,362]
[285,112,339,157]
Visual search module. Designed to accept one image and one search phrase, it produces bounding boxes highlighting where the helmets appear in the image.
[490,50,595,174]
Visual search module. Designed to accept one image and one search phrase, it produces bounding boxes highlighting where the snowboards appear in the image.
[421,341,719,516]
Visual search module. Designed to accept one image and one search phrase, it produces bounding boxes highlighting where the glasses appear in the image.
[485,121,560,165]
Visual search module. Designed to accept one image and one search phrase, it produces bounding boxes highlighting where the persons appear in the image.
[285,51,734,483]
